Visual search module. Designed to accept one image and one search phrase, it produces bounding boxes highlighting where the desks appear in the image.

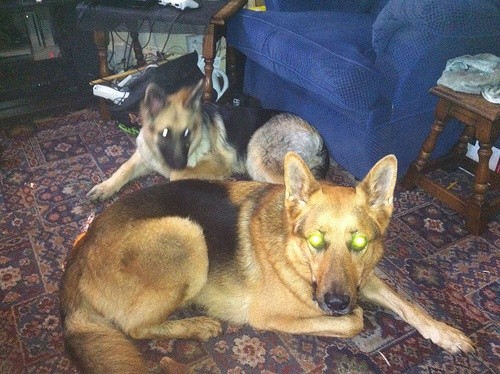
[404,68,500,236]
[75,0,228,111]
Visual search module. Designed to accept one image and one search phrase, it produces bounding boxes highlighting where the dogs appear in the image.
[60,78,477,374]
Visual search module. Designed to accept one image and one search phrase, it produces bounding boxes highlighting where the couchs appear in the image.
[223,0,500,192]
